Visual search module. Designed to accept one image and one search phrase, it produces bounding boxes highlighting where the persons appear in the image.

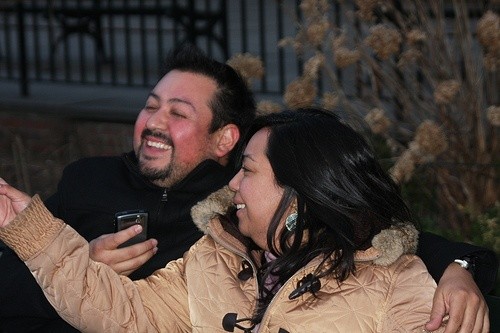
[0,108,449,333]
[0,40,500,333]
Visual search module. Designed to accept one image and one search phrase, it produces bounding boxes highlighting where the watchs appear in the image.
[450,255,475,272]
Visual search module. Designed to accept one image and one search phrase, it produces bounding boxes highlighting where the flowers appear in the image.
[228,0,500,234]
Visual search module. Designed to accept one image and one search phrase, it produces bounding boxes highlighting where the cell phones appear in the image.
[114,210,148,248]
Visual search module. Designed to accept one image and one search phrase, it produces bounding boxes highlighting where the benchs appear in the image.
[50,1,228,78]
